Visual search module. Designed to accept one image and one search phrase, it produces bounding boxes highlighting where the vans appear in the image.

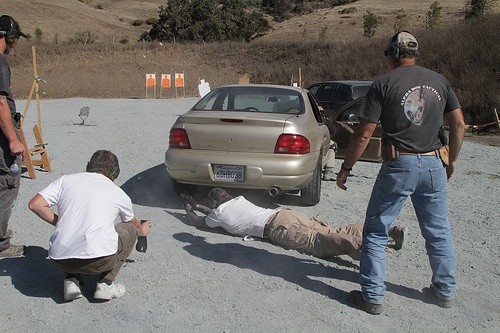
[303,79,374,121]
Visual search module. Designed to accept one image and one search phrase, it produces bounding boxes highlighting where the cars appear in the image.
[164,84,383,207]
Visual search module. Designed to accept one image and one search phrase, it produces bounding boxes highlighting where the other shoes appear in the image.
[348,290,382,314]
[391,225,408,250]
[422,286,452,308]
[322,169,338,181]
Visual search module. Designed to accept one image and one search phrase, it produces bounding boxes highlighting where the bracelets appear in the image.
[341,163,353,171]
[196,204,199,210]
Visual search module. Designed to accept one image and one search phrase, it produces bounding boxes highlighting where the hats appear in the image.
[0,15,28,41]
[208,187,231,206]
[386,30,418,54]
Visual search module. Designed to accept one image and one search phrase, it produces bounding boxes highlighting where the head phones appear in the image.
[6,16,16,44]
[86,154,120,182]
[387,30,411,61]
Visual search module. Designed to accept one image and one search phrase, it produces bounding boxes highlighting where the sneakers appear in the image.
[63,278,82,301]
[0,243,26,258]
[94,281,126,301]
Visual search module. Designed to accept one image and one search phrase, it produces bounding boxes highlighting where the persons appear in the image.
[183,188,405,261]
[336,31,467,316]
[29,150,154,301]
[0,14,28,258]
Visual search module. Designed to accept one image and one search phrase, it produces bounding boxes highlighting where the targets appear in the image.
[238,73,249,84]
[146,73,156,99]
[198,79,211,98]
[160,73,171,99]
[174,71,185,98]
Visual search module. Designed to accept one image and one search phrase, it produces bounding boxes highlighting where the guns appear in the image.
[176,192,192,203]
